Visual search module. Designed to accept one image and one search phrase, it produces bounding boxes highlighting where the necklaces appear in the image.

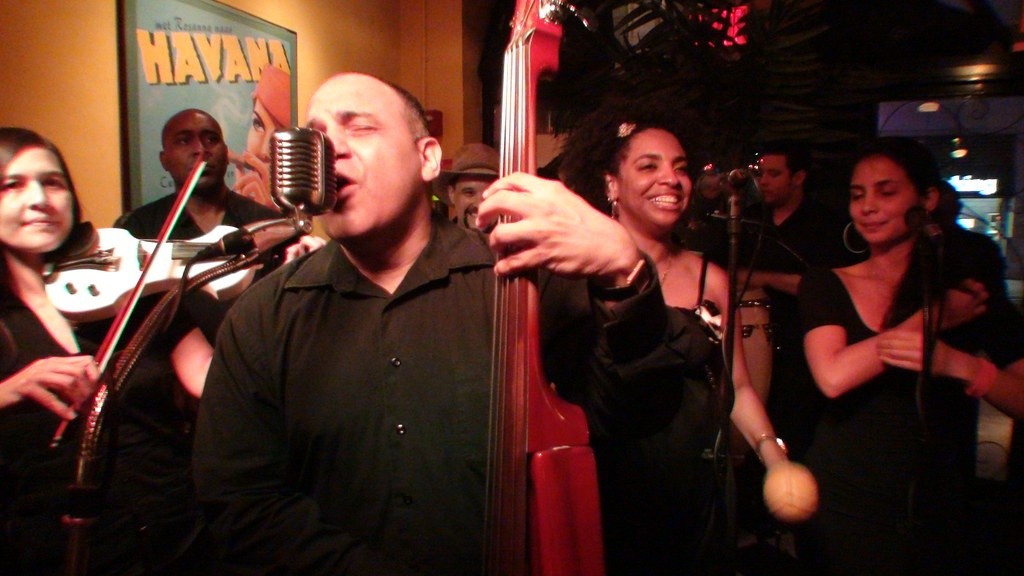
[660,251,676,284]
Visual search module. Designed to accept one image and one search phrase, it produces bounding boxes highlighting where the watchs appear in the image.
[598,254,651,302]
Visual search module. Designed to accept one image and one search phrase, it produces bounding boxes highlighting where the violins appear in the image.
[41,221,286,324]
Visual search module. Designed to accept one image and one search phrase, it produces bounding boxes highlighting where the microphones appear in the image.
[905,205,949,246]
[191,127,337,264]
[696,169,748,201]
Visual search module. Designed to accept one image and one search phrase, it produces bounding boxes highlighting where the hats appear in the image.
[429,143,498,204]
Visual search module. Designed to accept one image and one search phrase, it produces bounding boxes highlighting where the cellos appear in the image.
[477,0,609,576]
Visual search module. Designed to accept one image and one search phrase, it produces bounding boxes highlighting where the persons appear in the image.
[0,126,326,576]
[796,135,1024,576]
[433,142,500,231]
[554,96,817,576]
[721,136,835,300]
[193,69,669,576]
[122,108,307,352]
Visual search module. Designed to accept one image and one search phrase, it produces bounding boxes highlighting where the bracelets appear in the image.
[966,354,998,398]
[755,435,788,463]
